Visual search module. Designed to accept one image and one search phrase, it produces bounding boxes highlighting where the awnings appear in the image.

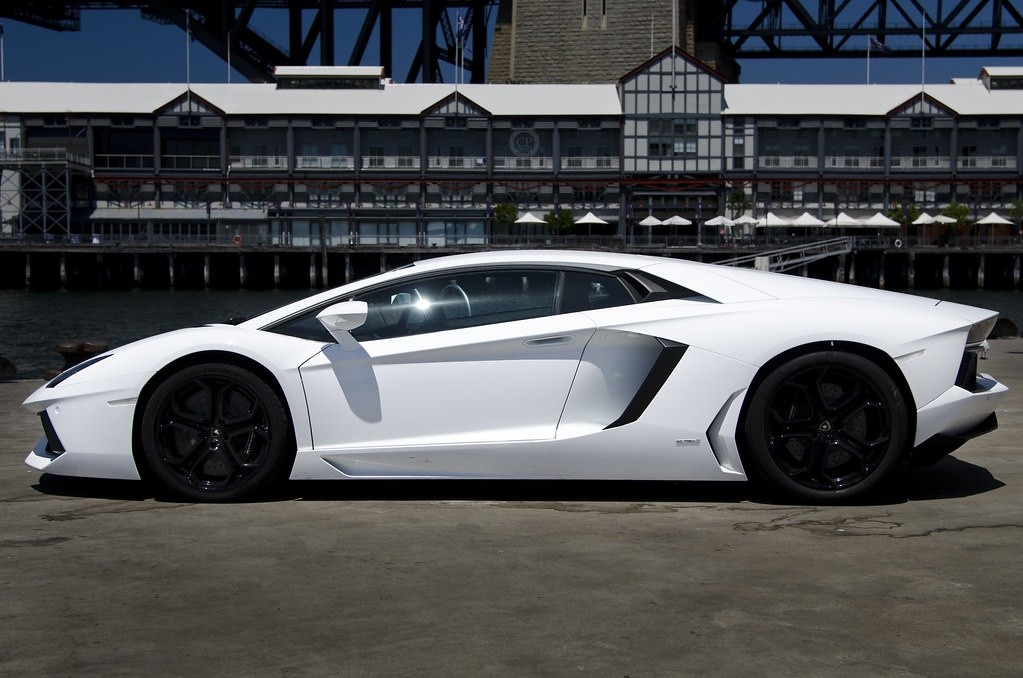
[91,208,268,219]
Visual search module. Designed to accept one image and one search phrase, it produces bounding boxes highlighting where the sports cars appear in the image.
[21,248,1011,506]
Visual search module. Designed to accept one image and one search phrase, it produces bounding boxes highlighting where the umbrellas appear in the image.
[514,212,548,233]
[639,216,662,234]
[705,215,736,226]
[973,212,1014,245]
[734,215,760,225]
[826,212,901,227]
[574,212,609,233]
[912,212,958,243]
[663,215,692,234]
[757,211,826,228]
